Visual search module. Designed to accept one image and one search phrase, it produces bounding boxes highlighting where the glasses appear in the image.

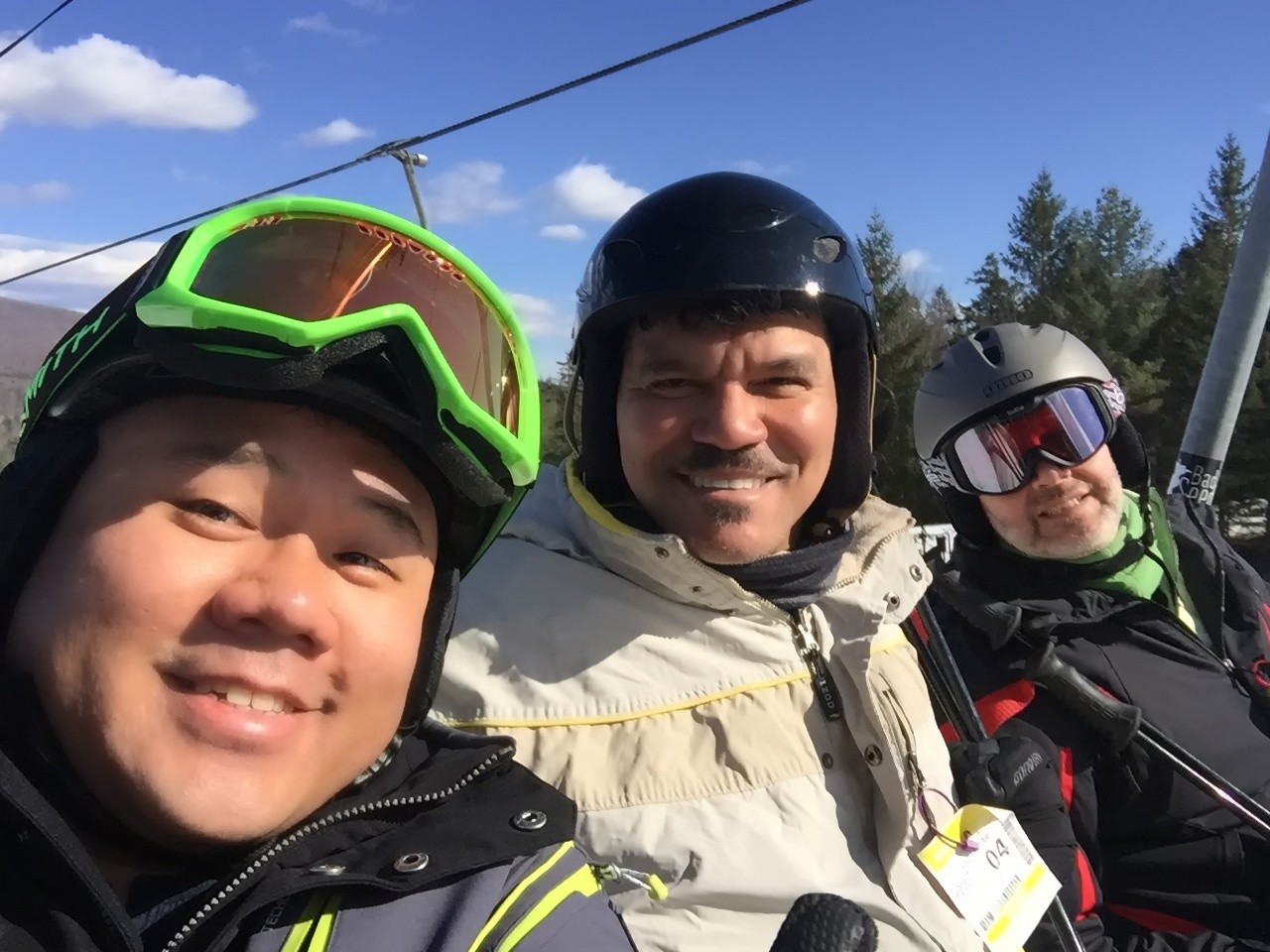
[940,383,1115,495]
[133,198,540,487]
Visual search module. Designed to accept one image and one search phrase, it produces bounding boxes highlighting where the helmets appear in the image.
[914,321,1149,541]
[0,258,501,734]
[573,171,877,523]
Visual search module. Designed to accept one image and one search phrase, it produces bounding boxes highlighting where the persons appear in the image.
[430,173,1078,952]
[0,197,648,952]
[900,322,1270,952]
